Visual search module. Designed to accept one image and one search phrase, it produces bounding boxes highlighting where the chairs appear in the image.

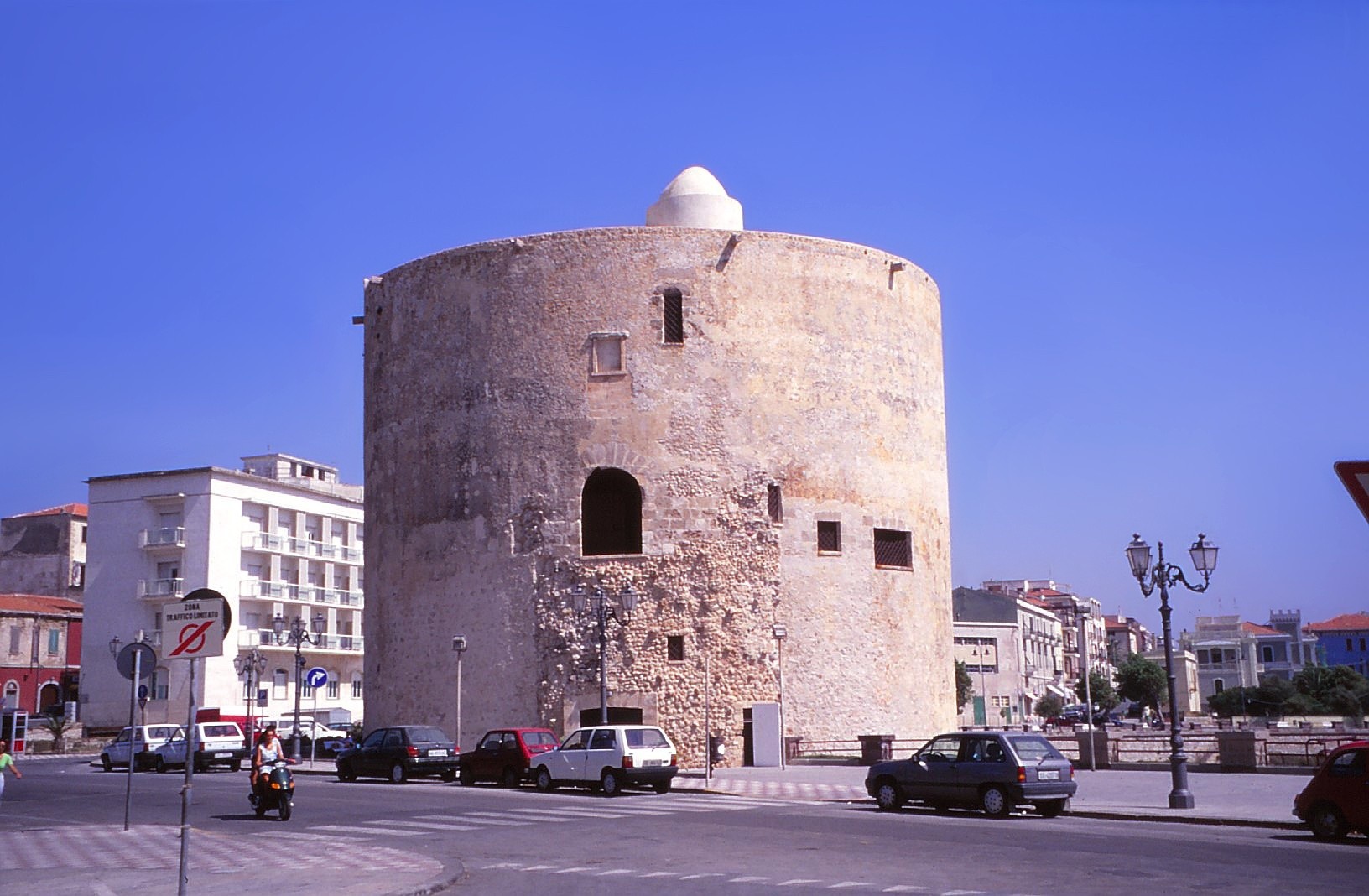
[389,736,401,746]
[486,741,516,749]
[597,738,612,749]
[987,743,1000,763]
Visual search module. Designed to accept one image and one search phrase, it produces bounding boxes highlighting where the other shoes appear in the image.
[288,800,295,807]
[253,795,260,809]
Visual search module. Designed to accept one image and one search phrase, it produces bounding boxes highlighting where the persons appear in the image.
[0,739,22,794]
[249,729,296,806]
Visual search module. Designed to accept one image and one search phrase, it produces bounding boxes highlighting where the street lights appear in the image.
[570,578,639,740]
[1125,530,1221,809]
[971,638,991,731]
[271,611,327,765]
[233,648,269,761]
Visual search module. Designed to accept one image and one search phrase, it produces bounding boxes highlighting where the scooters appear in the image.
[248,756,296,821]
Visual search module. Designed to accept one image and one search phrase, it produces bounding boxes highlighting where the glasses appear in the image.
[0,744,5,747]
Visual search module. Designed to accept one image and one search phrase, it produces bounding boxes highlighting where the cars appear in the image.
[154,722,247,773]
[100,723,185,773]
[1292,740,1369,844]
[459,727,564,789]
[1044,701,1165,730]
[528,725,681,797]
[335,725,461,785]
[865,730,1078,820]
